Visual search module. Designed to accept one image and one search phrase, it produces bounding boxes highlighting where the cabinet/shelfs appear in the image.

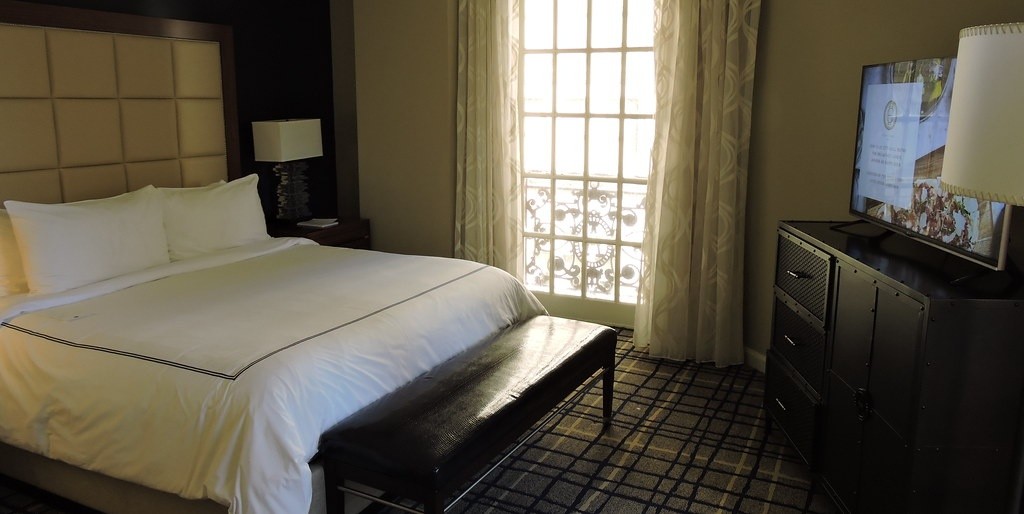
[764,221,1024,514]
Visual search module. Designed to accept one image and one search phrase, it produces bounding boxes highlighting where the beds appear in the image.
[0,1,549,514]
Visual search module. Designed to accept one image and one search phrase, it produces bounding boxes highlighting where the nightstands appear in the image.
[285,215,371,251]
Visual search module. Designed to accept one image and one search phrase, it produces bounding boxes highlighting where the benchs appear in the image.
[320,315,617,514]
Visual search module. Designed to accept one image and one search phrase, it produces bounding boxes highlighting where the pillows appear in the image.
[156,174,270,262]
[0,185,171,297]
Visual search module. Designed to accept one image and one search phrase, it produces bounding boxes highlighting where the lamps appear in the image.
[940,23,1024,207]
[252,118,323,226]
